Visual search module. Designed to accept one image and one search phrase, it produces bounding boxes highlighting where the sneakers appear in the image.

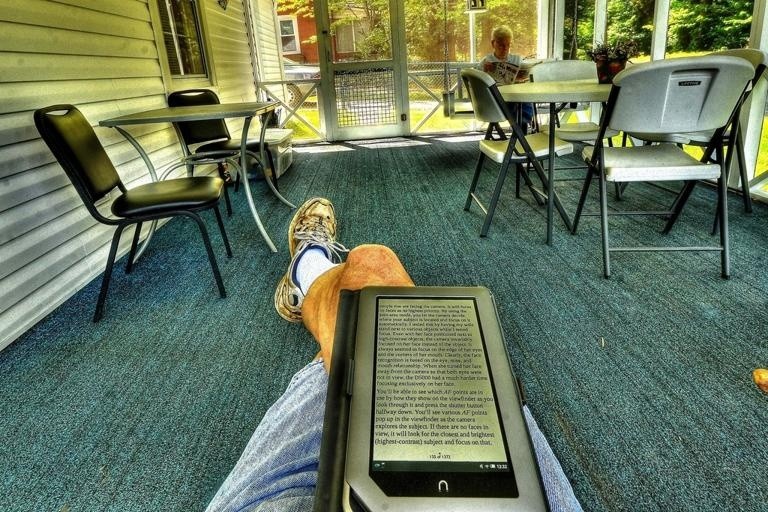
[274,197,337,322]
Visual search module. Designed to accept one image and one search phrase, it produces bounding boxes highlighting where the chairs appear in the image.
[570,54,755,278]
[32,103,236,323]
[165,87,301,214]
[529,58,629,199]
[616,47,765,235]
[461,65,581,240]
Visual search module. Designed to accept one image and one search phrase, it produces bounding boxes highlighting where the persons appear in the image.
[205,194,584,512]
[479,24,536,136]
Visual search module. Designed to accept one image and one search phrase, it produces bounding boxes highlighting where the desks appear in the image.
[495,77,617,248]
[97,101,298,264]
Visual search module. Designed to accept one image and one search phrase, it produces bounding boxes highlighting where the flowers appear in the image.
[583,32,641,69]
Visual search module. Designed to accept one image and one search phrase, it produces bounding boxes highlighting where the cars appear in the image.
[283,56,320,107]
